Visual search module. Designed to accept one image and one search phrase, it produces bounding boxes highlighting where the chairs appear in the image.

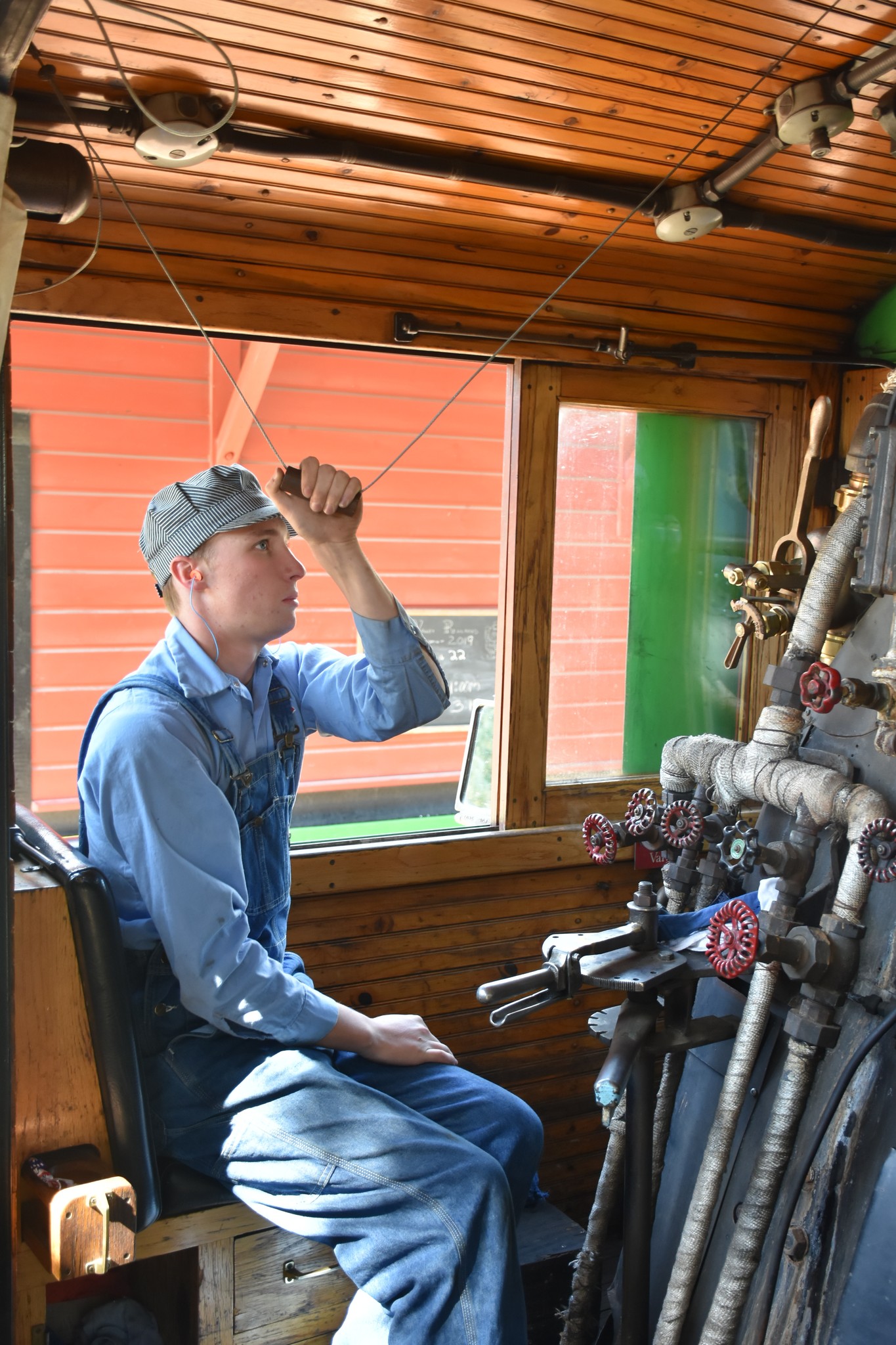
[15,802,359,1345]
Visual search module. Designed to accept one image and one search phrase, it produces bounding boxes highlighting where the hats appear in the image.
[137,462,300,598]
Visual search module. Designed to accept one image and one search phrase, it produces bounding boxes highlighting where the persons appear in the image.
[79,455,543,1345]
